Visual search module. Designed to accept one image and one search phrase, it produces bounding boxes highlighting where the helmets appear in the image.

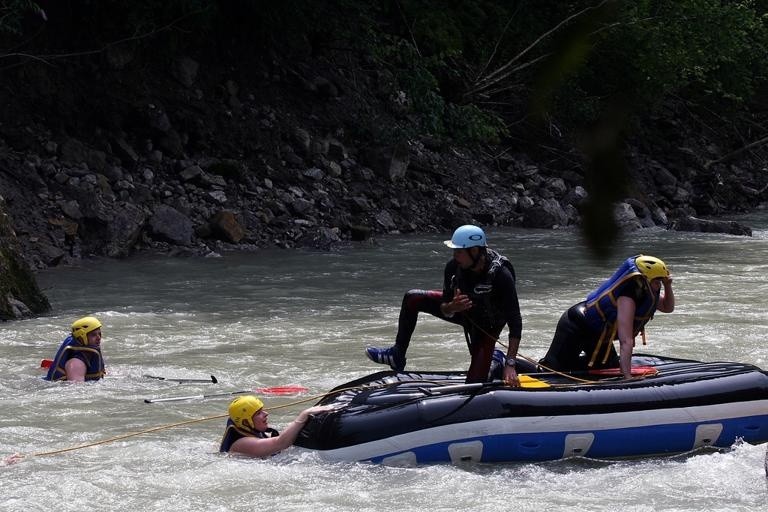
[634,254,670,289]
[228,394,263,427]
[71,316,102,337]
[443,223,490,249]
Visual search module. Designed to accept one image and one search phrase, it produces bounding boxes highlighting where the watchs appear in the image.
[504,357,517,367]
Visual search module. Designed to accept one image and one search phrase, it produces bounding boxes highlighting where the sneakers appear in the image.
[365,345,406,371]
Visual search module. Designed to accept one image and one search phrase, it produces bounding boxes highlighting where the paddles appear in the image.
[418,375,550,396]
[524,365,658,377]
[144,387,309,404]
[41,358,217,384]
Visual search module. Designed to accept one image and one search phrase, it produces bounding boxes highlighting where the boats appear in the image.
[292,353,768,471]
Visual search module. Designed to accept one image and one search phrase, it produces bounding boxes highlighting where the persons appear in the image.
[39,315,105,384]
[218,396,335,457]
[363,222,523,394]
[540,252,675,382]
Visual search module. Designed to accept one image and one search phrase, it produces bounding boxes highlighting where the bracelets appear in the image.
[294,415,308,424]
